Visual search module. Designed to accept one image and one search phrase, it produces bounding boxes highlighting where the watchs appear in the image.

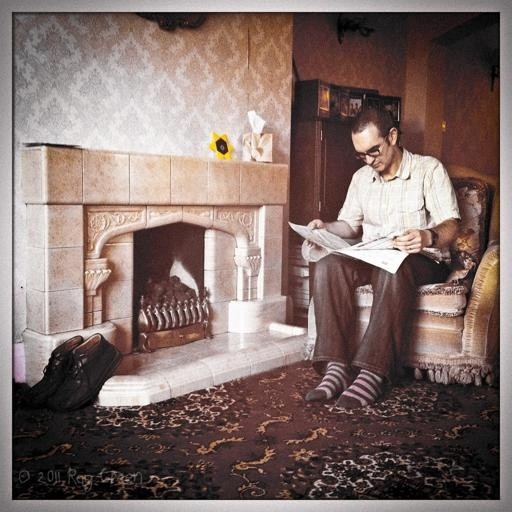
[424,228,439,248]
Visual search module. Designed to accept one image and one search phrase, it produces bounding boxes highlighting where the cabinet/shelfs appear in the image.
[288,79,403,328]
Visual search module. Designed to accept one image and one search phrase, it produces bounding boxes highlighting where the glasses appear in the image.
[353,138,386,161]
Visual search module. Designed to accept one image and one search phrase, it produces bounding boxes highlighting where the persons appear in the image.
[301,106,464,410]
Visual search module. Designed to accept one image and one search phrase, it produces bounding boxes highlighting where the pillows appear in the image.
[446,176,490,271]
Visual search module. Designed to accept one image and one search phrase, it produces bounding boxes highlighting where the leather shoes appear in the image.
[27,334,123,414]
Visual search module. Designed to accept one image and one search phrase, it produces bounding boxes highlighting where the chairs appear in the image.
[304,161,500,389]
